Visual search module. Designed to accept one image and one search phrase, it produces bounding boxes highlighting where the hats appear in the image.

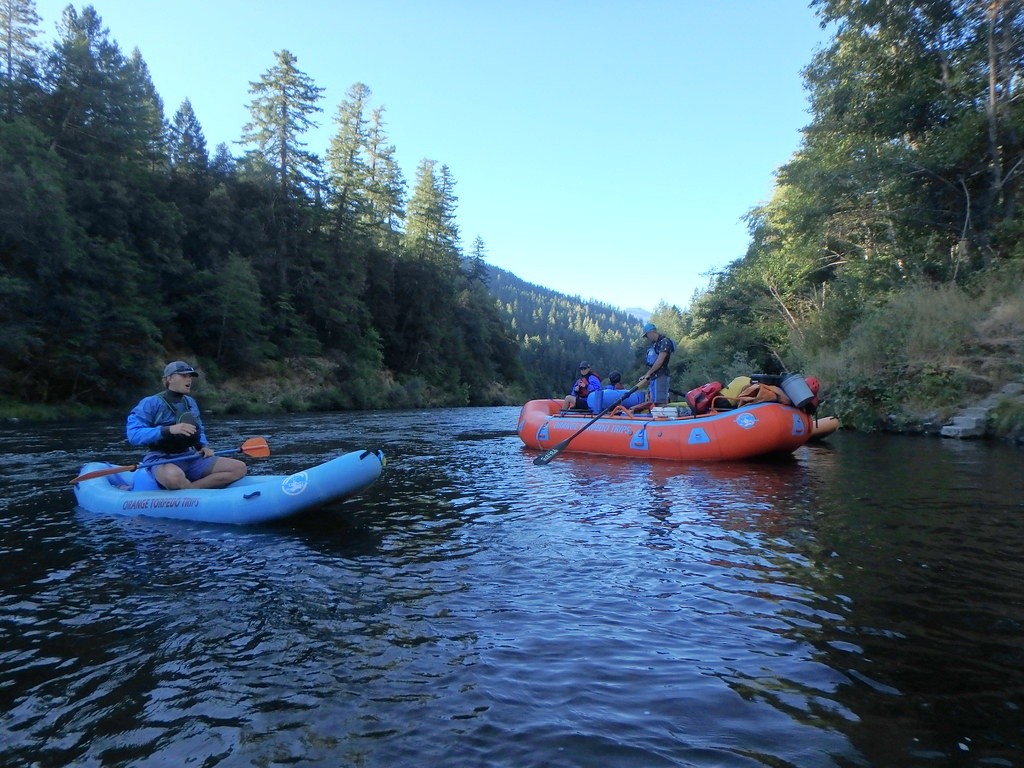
[608,371,621,386]
[579,361,589,367]
[162,360,199,378]
[640,323,656,338]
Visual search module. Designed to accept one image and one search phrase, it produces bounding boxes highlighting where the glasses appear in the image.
[642,334,647,338]
[580,368,587,370]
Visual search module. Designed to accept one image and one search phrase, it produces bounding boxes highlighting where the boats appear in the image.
[515,398,814,459]
[69,449,387,523]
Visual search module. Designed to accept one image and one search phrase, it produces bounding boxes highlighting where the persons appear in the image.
[562,361,602,410]
[604,372,623,415]
[640,323,672,403]
[127,362,247,490]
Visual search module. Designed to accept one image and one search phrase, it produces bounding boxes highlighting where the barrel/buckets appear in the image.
[650,406,678,417]
[781,371,814,407]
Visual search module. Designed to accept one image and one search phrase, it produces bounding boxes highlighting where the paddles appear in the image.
[67,436,271,484]
[531,376,651,466]
[668,388,688,401]
